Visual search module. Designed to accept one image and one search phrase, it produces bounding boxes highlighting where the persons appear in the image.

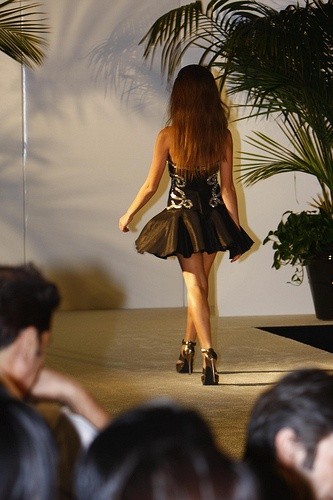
[118,64,254,385]
[0,262,333,500]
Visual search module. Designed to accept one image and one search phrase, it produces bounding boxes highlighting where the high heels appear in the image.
[176,339,196,375]
[200,348,220,386]
